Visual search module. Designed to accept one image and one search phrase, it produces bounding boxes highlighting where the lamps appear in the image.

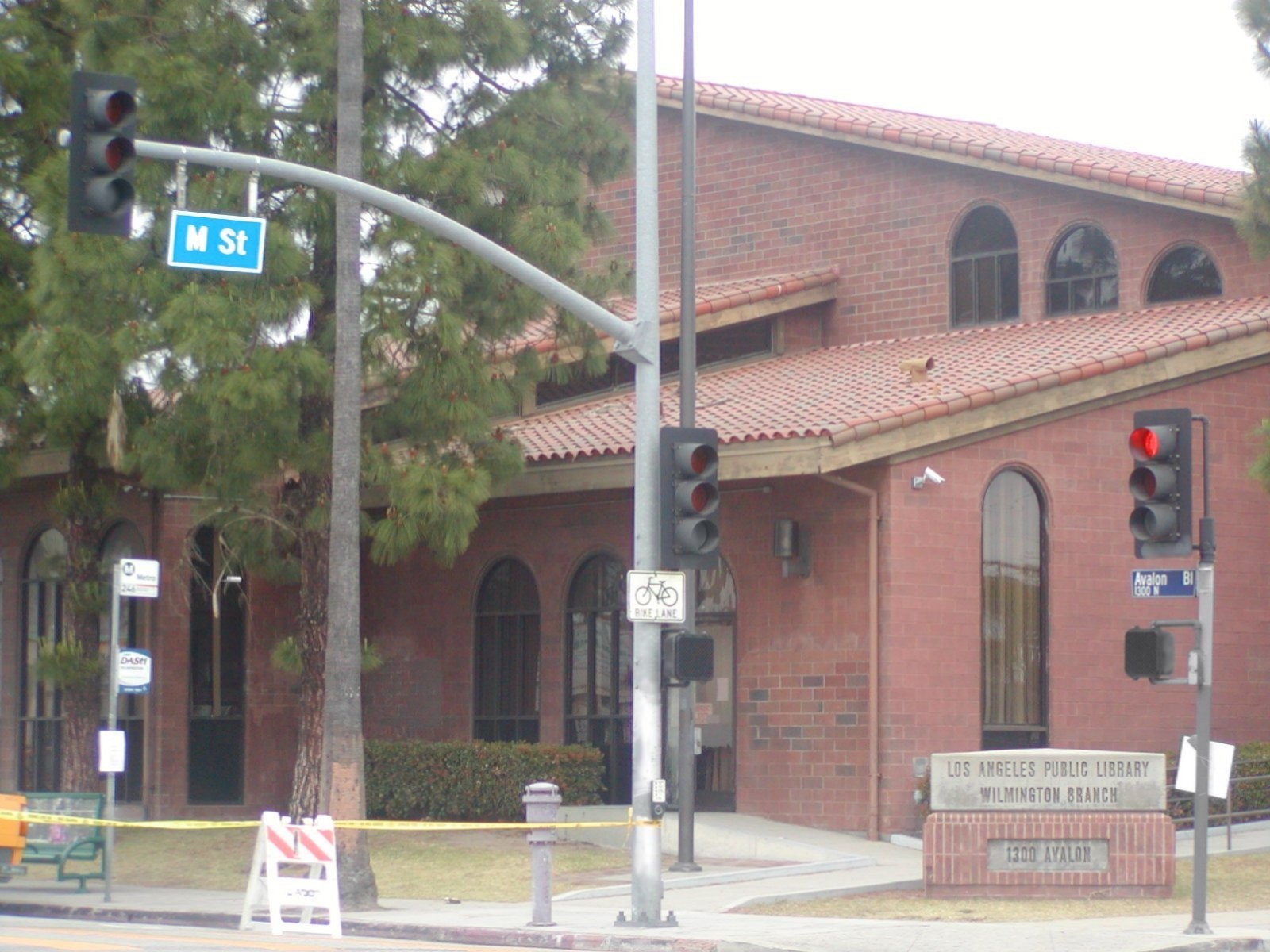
[774,520,807,580]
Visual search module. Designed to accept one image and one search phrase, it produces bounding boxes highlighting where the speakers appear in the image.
[1124,629,1175,680]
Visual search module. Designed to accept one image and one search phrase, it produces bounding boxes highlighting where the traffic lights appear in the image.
[66,70,139,238]
[1123,625,1175,680]
[662,632,715,683]
[1127,408,1192,560]
[660,426,721,569]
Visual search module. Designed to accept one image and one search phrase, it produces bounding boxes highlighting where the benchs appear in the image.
[20,792,105,894]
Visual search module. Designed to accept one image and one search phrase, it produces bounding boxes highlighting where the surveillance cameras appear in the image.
[923,467,945,484]
